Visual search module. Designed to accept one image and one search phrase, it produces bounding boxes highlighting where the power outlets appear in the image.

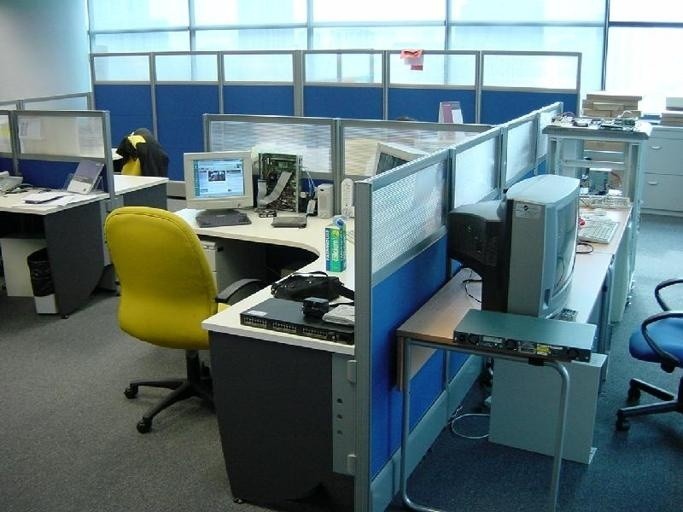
[586,194,629,209]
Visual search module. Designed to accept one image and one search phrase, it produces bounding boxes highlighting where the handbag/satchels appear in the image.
[271,270,355,308]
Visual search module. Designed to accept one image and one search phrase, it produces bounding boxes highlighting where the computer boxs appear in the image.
[488,352,608,464]
[200,240,249,294]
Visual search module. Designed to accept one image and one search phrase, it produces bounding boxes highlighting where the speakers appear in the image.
[317,183,334,219]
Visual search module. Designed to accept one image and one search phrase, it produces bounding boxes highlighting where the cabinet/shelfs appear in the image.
[641,126,682,216]
[541,125,648,323]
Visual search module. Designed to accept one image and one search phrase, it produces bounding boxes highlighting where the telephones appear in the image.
[0,171,24,194]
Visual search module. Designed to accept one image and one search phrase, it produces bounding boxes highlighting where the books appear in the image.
[659,109,683,126]
[67,160,105,194]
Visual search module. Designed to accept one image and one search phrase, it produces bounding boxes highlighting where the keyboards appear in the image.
[577,218,619,244]
[195,213,250,228]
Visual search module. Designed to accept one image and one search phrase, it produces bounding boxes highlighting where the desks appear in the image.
[394,201,634,512]
[0,186,110,319]
[82,148,169,213]
[171,205,359,512]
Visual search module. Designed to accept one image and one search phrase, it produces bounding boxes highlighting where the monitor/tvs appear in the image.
[447,174,580,319]
[183,151,254,216]
[372,142,430,178]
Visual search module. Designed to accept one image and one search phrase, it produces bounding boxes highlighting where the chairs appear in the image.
[614,275,682,434]
[104,207,265,434]
[122,128,167,211]
